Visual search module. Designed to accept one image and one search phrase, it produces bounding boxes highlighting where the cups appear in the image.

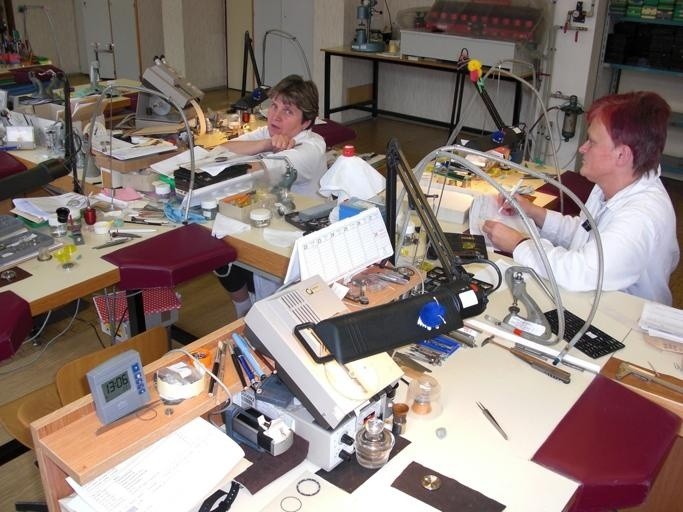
[405,374,445,419]
[83,207,97,225]
[389,39,400,52]
[243,112,250,122]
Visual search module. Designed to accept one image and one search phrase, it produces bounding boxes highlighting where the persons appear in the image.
[480,89,681,311]
[204,74,336,321]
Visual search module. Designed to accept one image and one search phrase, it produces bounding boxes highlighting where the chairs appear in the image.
[1,322,167,471]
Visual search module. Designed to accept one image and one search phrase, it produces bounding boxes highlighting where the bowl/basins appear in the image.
[57,244,76,270]
[94,220,110,235]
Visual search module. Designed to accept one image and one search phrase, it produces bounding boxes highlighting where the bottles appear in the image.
[392,404,409,435]
[200,197,219,220]
[155,183,171,209]
[56,216,67,237]
[250,208,271,227]
[354,418,396,470]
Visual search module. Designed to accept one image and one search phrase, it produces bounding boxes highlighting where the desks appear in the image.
[30,146,683,512]
[318,42,539,145]
[0,76,385,357]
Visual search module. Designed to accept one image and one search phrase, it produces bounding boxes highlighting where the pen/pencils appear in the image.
[208,332,278,397]
[498,179,523,213]
